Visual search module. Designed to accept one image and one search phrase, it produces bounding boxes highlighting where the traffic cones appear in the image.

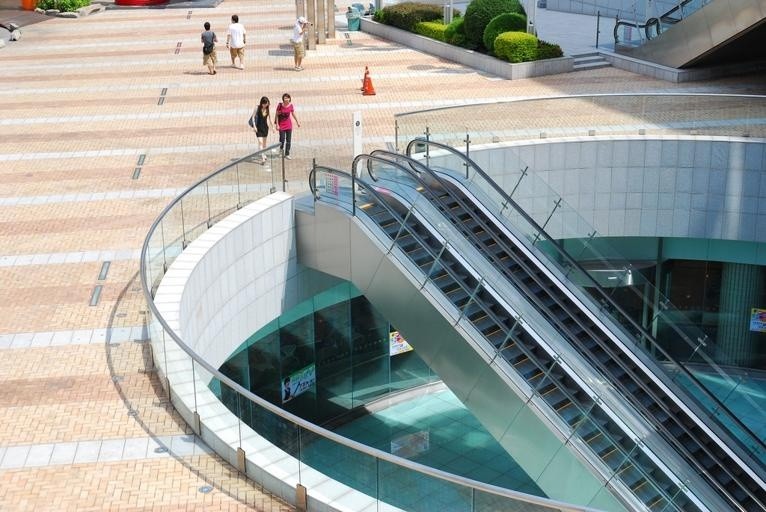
[360,65,377,95]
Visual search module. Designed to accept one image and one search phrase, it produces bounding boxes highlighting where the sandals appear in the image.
[262,157,268,164]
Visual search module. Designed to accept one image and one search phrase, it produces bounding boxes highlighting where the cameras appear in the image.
[306,22,313,27]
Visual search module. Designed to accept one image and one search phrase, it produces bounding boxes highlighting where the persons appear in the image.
[226,14,247,70]
[201,22,219,75]
[250,96,273,164]
[275,93,300,160]
[284,377,292,400]
[291,16,310,71]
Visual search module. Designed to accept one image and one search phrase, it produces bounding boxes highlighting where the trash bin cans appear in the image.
[346,7,360,31]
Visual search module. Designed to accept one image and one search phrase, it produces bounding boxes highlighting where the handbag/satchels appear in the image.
[248,115,257,127]
[203,45,212,54]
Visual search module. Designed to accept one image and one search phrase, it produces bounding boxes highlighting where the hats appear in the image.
[298,17,307,24]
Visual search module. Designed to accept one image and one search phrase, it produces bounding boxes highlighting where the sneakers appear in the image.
[231,64,244,69]
[279,150,291,161]
[295,66,303,70]
